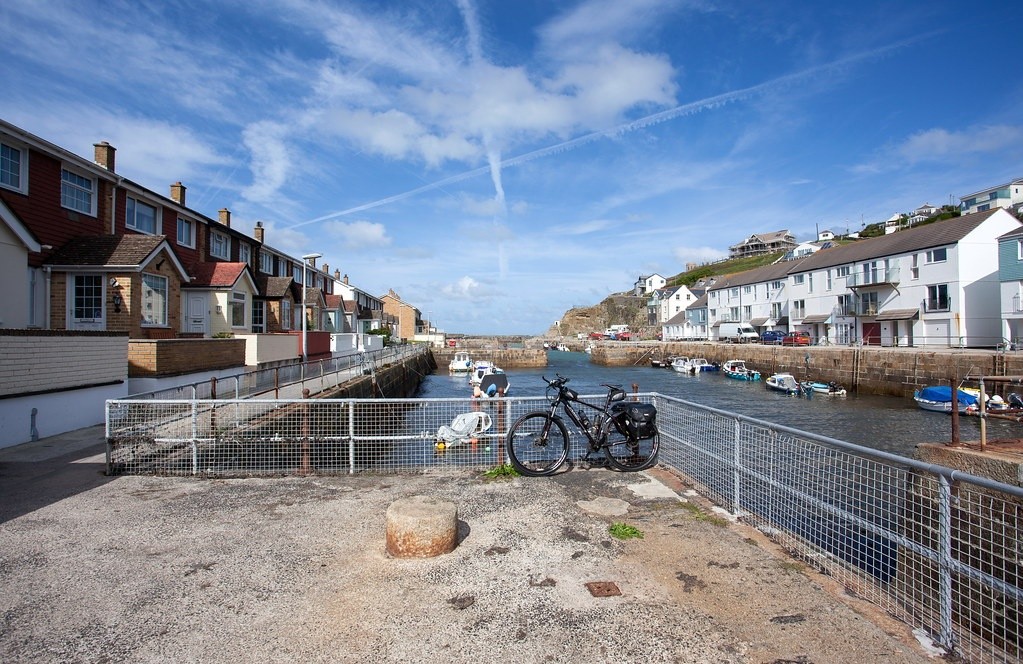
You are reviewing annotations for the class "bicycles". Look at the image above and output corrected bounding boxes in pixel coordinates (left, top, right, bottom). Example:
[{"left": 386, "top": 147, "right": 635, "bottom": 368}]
[{"left": 507, "top": 376, "right": 659, "bottom": 476}]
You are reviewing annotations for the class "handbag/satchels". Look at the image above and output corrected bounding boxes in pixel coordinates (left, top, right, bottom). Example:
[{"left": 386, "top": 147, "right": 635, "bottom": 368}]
[
  {"left": 626, "top": 403, "right": 658, "bottom": 442},
  {"left": 610, "top": 401, "right": 641, "bottom": 436}
]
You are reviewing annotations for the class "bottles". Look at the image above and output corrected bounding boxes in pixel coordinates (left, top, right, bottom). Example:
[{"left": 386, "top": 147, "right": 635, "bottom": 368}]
[
  {"left": 591, "top": 414, "right": 601, "bottom": 437},
  {"left": 577, "top": 409, "right": 591, "bottom": 430}
]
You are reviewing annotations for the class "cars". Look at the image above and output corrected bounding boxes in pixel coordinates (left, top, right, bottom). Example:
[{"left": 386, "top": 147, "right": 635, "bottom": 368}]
[
  {"left": 780, "top": 332, "right": 812, "bottom": 346},
  {"left": 760, "top": 331, "right": 787, "bottom": 345}
]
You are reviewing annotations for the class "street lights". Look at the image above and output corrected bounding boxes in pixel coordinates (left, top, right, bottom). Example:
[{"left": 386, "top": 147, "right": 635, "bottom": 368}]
[{"left": 303, "top": 253, "right": 322, "bottom": 360}]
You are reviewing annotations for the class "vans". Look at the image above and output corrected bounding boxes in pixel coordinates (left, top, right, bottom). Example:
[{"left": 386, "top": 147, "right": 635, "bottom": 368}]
[{"left": 718, "top": 323, "right": 759, "bottom": 343}]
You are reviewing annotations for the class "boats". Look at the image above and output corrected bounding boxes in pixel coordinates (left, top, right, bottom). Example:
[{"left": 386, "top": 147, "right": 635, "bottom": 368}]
[
  {"left": 475, "top": 368, "right": 509, "bottom": 407},
  {"left": 690, "top": 358, "right": 720, "bottom": 372},
  {"left": 766, "top": 372, "right": 801, "bottom": 396},
  {"left": 723, "top": 359, "right": 761, "bottom": 381},
  {"left": 648, "top": 357, "right": 671, "bottom": 369},
  {"left": 809, "top": 381, "right": 847, "bottom": 394},
  {"left": 469, "top": 360, "right": 503, "bottom": 387},
  {"left": 671, "top": 356, "right": 700, "bottom": 374},
  {"left": 448, "top": 351, "right": 473, "bottom": 371},
  {"left": 913, "top": 386, "right": 990, "bottom": 414}
]
